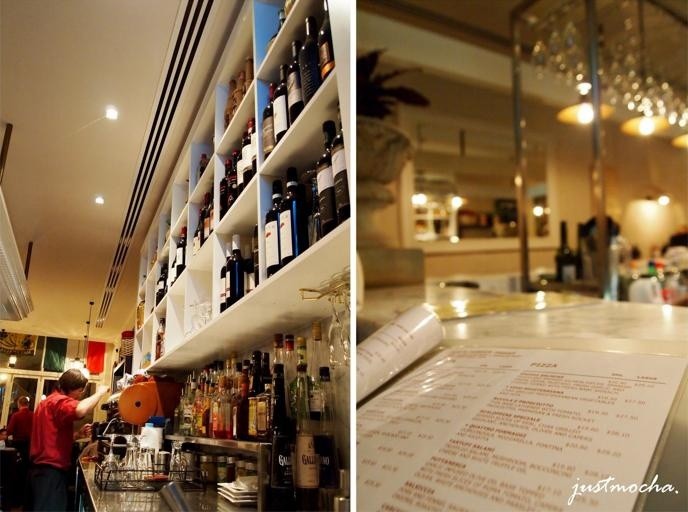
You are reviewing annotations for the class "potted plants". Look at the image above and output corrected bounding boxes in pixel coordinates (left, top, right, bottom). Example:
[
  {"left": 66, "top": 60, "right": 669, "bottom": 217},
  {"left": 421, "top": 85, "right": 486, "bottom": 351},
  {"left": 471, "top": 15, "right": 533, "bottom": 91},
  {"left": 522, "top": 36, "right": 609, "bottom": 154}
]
[{"left": 356, "top": 48, "right": 430, "bottom": 288}]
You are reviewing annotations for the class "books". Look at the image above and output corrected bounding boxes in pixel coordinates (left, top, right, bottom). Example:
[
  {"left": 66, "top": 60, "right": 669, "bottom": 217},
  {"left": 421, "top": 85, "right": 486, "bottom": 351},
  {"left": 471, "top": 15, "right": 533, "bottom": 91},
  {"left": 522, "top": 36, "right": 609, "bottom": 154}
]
[{"left": 357, "top": 301, "right": 688, "bottom": 511}]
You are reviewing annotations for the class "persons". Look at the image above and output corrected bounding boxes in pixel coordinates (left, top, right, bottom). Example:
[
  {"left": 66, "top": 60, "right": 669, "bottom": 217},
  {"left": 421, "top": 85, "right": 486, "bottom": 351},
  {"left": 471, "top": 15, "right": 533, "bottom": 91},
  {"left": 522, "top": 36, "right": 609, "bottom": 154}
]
[
  {"left": 29, "top": 367, "right": 111, "bottom": 512},
  {"left": 6, "top": 397, "right": 36, "bottom": 508},
  {"left": 0, "top": 428, "right": 13, "bottom": 448}
]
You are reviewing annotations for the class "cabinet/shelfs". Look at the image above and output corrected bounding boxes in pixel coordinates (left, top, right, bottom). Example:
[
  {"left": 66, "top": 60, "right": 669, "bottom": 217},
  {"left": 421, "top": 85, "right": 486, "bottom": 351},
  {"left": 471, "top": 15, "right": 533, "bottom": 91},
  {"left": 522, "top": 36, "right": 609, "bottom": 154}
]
[{"left": 111, "top": 0, "right": 351, "bottom": 403}]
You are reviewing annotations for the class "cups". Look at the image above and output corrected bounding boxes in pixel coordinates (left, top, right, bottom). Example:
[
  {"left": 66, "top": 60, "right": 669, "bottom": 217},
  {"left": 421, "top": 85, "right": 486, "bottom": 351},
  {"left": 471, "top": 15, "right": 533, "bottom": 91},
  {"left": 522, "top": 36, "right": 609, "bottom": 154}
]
[{"left": 123, "top": 445, "right": 153, "bottom": 470}]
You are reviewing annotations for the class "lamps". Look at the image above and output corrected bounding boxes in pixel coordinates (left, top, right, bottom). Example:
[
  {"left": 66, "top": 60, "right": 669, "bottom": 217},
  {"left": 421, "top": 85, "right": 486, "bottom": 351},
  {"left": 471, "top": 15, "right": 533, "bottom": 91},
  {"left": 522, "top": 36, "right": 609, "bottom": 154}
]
[
  {"left": 9, "top": 334, "right": 17, "bottom": 368},
  {"left": 557, "top": 0, "right": 688, "bottom": 149}
]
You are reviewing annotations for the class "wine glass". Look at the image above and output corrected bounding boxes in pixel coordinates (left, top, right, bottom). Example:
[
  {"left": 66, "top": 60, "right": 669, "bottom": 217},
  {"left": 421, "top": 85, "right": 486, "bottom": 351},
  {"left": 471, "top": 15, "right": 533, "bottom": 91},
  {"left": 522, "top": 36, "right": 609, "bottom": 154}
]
[
  {"left": 528, "top": 5, "right": 687, "bottom": 126},
  {"left": 328, "top": 282, "right": 351, "bottom": 369}
]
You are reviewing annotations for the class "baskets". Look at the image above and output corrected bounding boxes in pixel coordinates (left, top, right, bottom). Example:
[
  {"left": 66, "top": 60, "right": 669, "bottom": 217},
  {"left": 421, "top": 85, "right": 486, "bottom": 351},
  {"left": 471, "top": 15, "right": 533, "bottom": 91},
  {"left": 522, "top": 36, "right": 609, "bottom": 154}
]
[{"left": 118, "top": 337, "right": 135, "bottom": 357}]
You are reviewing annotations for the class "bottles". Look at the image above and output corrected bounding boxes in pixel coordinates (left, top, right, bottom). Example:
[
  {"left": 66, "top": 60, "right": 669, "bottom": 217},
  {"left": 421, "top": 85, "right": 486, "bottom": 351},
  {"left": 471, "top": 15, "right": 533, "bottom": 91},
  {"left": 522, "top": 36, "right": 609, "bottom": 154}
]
[
  {"left": 154, "top": 152, "right": 211, "bottom": 362},
  {"left": 556, "top": 221, "right": 578, "bottom": 281},
  {"left": 221, "top": 2, "right": 349, "bottom": 312},
  {"left": 174, "top": 321, "right": 336, "bottom": 508}
]
